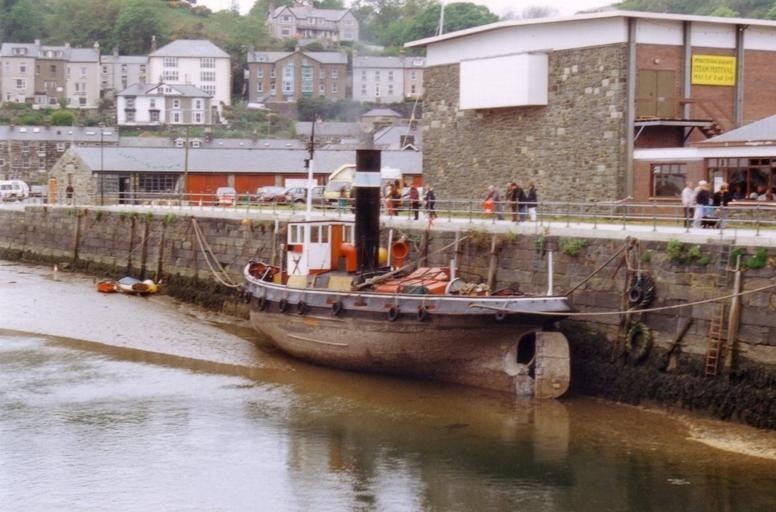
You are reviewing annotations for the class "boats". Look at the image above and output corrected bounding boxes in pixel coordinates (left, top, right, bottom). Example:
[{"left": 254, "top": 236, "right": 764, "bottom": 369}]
[
  {"left": 98, "top": 275, "right": 159, "bottom": 295},
  {"left": 241, "top": 149, "right": 574, "bottom": 397}
]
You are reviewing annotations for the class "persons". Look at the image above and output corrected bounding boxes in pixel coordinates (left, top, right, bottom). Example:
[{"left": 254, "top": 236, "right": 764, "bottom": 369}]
[
  {"left": 381, "top": 178, "right": 403, "bottom": 217},
  {"left": 680, "top": 179, "right": 734, "bottom": 235},
  {"left": 731, "top": 184, "right": 746, "bottom": 199},
  {"left": 407, "top": 182, "right": 421, "bottom": 221},
  {"left": 505, "top": 182, "right": 518, "bottom": 222},
  {"left": 421, "top": 184, "right": 438, "bottom": 220},
  {"left": 337, "top": 185, "right": 349, "bottom": 214},
  {"left": 348, "top": 186, "right": 358, "bottom": 214},
  {"left": 483, "top": 185, "right": 505, "bottom": 221},
  {"left": 506, "top": 183, "right": 528, "bottom": 223},
  {"left": 525, "top": 180, "right": 540, "bottom": 222},
  {"left": 755, "top": 183, "right": 768, "bottom": 196}
]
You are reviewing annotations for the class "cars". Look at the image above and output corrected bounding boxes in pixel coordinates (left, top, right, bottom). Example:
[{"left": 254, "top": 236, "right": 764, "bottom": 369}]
[
  {"left": 214, "top": 165, "right": 425, "bottom": 209},
  {"left": 1, "top": 179, "right": 47, "bottom": 202}
]
